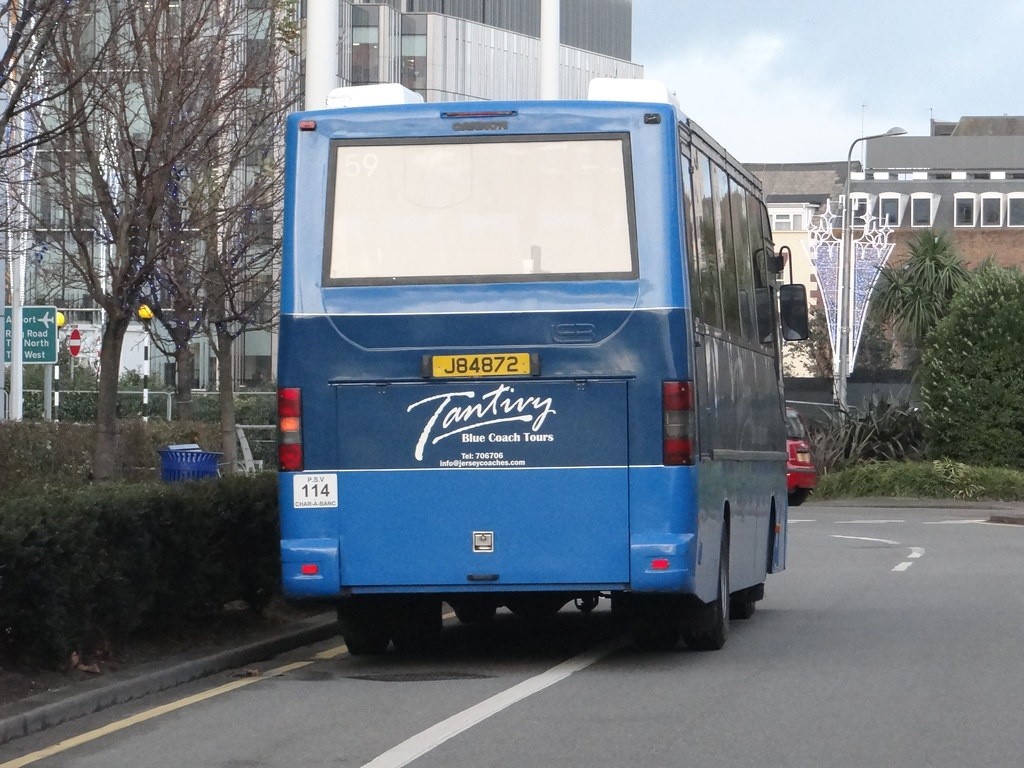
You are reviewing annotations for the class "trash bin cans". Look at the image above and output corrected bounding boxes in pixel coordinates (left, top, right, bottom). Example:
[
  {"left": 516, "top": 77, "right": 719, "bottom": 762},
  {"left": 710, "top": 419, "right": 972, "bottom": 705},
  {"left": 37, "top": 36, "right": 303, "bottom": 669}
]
[{"left": 154, "top": 448, "right": 224, "bottom": 484}]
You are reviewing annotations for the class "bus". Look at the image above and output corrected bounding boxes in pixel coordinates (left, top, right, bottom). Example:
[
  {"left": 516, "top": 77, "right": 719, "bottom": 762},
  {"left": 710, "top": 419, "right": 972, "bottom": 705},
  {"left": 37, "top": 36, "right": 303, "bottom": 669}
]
[{"left": 278, "top": 84, "right": 808, "bottom": 650}]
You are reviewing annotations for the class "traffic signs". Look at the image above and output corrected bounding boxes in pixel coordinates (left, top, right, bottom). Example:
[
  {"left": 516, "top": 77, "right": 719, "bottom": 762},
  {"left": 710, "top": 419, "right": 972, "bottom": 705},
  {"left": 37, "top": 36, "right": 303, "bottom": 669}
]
[{"left": 2, "top": 307, "right": 57, "bottom": 363}]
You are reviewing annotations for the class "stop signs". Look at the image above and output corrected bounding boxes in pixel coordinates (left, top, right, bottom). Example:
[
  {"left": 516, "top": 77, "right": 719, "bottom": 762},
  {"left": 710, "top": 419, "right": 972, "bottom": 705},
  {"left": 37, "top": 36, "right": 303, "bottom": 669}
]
[{"left": 69, "top": 329, "right": 81, "bottom": 356}]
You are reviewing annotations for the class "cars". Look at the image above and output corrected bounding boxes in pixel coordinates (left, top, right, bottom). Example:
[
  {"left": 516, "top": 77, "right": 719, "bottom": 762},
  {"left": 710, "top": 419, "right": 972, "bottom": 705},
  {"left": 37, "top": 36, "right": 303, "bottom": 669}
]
[{"left": 777, "top": 437, "right": 816, "bottom": 505}]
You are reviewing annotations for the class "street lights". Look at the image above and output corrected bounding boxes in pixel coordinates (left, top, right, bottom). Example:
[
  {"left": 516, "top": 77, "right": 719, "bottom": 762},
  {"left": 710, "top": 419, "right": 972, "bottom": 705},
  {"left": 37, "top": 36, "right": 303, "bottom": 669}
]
[
  {"left": 838, "top": 126, "right": 909, "bottom": 432},
  {"left": 54, "top": 312, "right": 65, "bottom": 425},
  {"left": 138, "top": 303, "right": 154, "bottom": 424}
]
